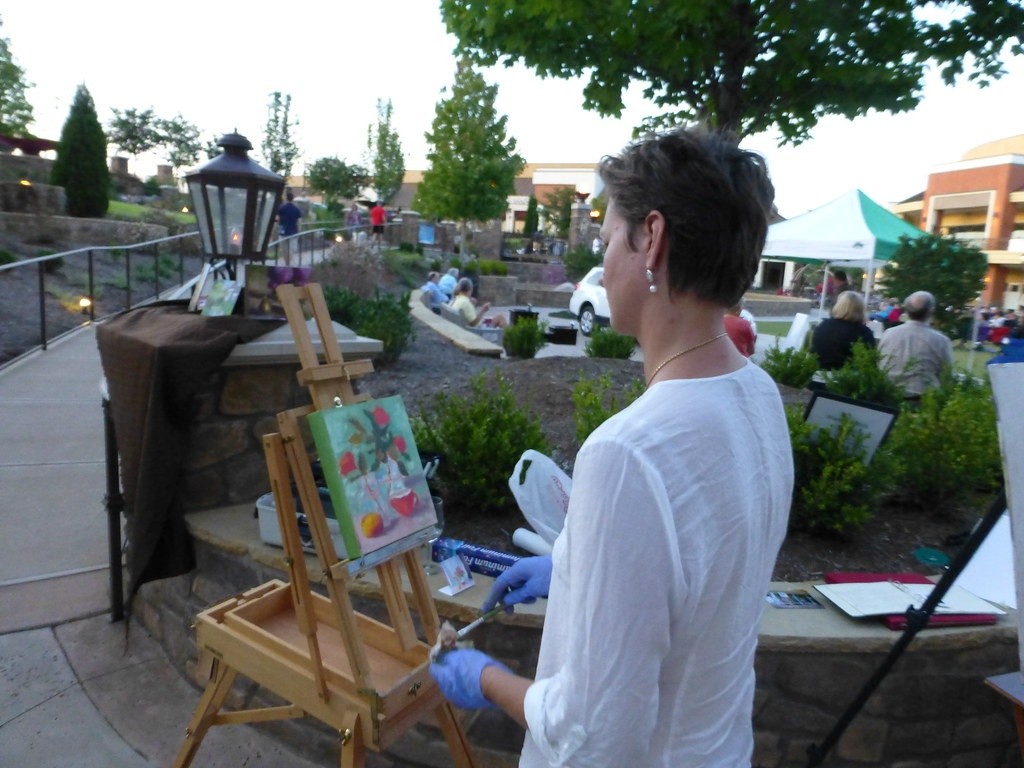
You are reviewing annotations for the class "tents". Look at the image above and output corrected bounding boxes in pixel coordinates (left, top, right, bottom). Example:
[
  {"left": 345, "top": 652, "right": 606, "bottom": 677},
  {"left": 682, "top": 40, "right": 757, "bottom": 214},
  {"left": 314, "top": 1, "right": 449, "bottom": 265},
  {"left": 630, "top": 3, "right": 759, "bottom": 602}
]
[{"left": 762, "top": 189, "right": 982, "bottom": 371}]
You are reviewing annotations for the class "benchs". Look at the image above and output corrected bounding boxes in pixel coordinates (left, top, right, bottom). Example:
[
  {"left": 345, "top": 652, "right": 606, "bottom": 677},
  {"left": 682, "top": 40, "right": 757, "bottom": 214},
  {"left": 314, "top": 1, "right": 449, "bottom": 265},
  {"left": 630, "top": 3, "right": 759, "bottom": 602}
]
[
  {"left": 421, "top": 288, "right": 444, "bottom": 315},
  {"left": 440, "top": 301, "right": 504, "bottom": 344}
]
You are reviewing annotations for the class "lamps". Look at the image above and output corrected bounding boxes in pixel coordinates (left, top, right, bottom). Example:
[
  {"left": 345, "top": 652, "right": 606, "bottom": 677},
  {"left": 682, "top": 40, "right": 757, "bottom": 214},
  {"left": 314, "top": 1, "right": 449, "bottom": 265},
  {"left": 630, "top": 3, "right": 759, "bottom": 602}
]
[
  {"left": 184, "top": 129, "right": 287, "bottom": 274},
  {"left": 80, "top": 298, "right": 91, "bottom": 313}
]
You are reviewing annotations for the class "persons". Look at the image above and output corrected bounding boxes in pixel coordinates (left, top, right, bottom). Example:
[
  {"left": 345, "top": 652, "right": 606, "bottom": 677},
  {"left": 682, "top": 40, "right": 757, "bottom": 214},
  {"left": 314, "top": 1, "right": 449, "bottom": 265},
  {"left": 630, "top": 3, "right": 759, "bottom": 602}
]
[
  {"left": 422, "top": 268, "right": 507, "bottom": 337},
  {"left": 349, "top": 204, "right": 361, "bottom": 240},
  {"left": 370, "top": 201, "right": 386, "bottom": 250},
  {"left": 980, "top": 305, "right": 1024, "bottom": 341},
  {"left": 275, "top": 193, "right": 302, "bottom": 266},
  {"left": 810, "top": 272, "right": 876, "bottom": 372},
  {"left": 725, "top": 300, "right": 755, "bottom": 358},
  {"left": 877, "top": 292, "right": 953, "bottom": 410},
  {"left": 868, "top": 298, "right": 902, "bottom": 323},
  {"left": 431, "top": 130, "right": 795, "bottom": 768}
]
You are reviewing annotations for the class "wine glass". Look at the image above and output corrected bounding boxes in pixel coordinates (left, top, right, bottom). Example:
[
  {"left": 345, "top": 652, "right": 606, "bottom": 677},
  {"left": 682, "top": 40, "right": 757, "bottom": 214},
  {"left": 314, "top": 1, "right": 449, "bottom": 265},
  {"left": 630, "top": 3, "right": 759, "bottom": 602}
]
[{"left": 419, "top": 496, "right": 444, "bottom": 576}]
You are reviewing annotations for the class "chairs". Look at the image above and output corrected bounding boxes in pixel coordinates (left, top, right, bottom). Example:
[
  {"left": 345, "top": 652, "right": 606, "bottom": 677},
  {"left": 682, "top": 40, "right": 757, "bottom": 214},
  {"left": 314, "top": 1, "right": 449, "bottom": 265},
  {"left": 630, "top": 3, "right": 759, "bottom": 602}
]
[
  {"left": 992, "top": 326, "right": 1010, "bottom": 343},
  {"left": 972, "top": 326, "right": 990, "bottom": 351}
]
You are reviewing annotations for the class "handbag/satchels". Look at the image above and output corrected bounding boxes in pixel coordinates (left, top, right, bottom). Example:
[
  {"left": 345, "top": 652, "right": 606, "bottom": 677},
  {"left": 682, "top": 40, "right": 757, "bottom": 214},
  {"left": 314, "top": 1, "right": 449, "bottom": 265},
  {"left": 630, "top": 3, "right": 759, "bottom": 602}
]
[{"left": 506, "top": 449, "right": 569, "bottom": 545}]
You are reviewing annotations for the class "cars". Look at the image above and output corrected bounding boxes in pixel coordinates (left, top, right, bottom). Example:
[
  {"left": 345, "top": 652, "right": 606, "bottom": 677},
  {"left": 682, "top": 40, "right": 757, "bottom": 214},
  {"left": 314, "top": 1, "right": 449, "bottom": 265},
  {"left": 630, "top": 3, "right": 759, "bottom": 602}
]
[{"left": 569, "top": 266, "right": 757, "bottom": 336}]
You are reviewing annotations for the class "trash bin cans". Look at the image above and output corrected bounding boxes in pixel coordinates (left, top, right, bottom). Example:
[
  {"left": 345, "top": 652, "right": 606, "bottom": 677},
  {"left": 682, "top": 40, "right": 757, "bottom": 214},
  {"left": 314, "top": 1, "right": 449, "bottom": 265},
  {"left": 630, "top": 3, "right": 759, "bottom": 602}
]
[{"left": 509, "top": 309, "right": 539, "bottom": 325}]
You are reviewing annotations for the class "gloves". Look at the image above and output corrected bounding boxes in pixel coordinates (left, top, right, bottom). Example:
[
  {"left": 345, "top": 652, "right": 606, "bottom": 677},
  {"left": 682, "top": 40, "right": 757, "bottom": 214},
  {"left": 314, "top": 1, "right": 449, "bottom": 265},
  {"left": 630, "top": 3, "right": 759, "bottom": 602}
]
[
  {"left": 429, "top": 649, "right": 514, "bottom": 710},
  {"left": 482, "top": 556, "right": 552, "bottom": 614}
]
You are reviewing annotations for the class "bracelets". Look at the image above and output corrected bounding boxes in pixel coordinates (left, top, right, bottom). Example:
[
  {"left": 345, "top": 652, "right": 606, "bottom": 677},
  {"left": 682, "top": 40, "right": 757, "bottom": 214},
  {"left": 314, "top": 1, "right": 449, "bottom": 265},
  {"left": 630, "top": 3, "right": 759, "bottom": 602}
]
[{"left": 646, "top": 332, "right": 728, "bottom": 387}]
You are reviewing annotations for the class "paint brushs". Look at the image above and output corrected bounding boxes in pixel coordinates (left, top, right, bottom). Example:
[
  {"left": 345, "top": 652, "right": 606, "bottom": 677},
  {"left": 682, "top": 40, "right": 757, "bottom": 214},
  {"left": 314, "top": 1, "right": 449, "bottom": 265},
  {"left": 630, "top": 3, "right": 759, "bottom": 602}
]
[{"left": 456, "top": 605, "right": 506, "bottom": 638}]
